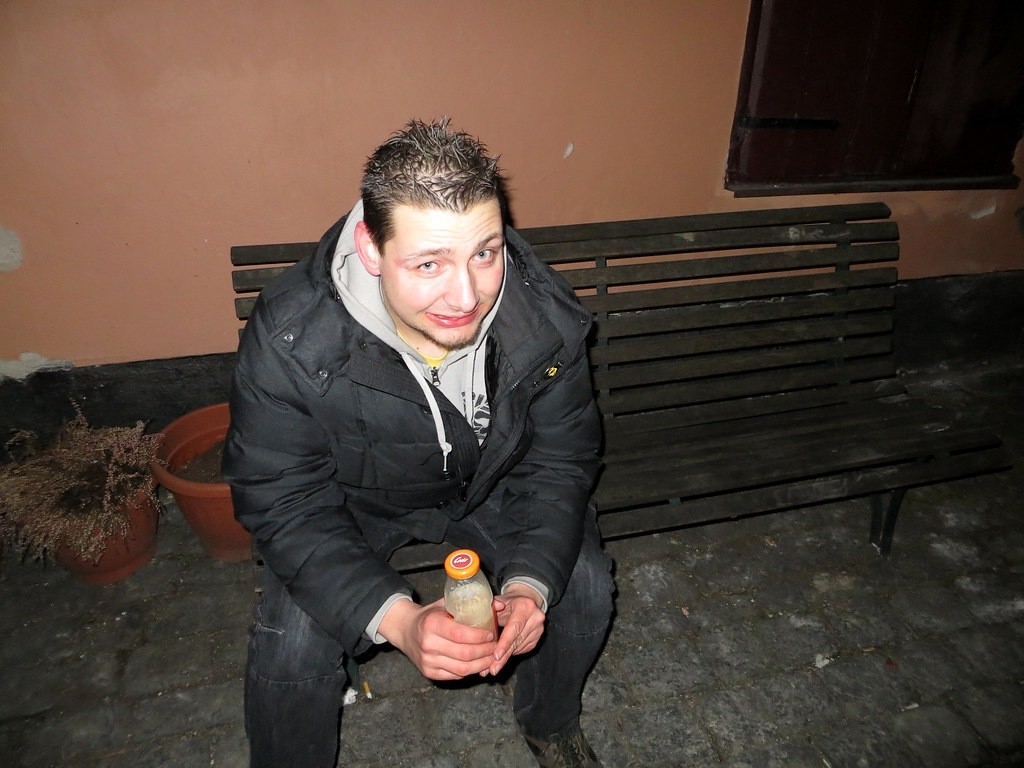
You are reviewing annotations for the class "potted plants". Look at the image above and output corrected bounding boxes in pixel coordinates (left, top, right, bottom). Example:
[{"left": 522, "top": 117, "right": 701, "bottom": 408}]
[{"left": 0, "top": 395, "right": 165, "bottom": 585}]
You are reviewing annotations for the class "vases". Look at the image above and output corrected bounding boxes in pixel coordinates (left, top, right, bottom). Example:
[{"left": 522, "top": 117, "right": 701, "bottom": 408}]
[{"left": 149, "top": 403, "right": 253, "bottom": 563}]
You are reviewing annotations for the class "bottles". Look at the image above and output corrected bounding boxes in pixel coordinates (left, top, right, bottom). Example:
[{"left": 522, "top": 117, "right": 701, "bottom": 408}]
[{"left": 443, "top": 549, "right": 500, "bottom": 643}]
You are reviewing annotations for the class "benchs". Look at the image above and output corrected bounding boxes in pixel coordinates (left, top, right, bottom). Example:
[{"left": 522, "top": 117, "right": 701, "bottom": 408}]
[{"left": 229, "top": 203, "right": 1015, "bottom": 717}]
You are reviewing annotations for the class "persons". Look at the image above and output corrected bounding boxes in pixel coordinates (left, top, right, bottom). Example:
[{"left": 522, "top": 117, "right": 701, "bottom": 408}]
[{"left": 221, "top": 113, "right": 614, "bottom": 768}]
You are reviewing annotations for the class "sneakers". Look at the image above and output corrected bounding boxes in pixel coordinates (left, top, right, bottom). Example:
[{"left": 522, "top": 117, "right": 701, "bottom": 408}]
[{"left": 520, "top": 723, "right": 604, "bottom": 768}]
